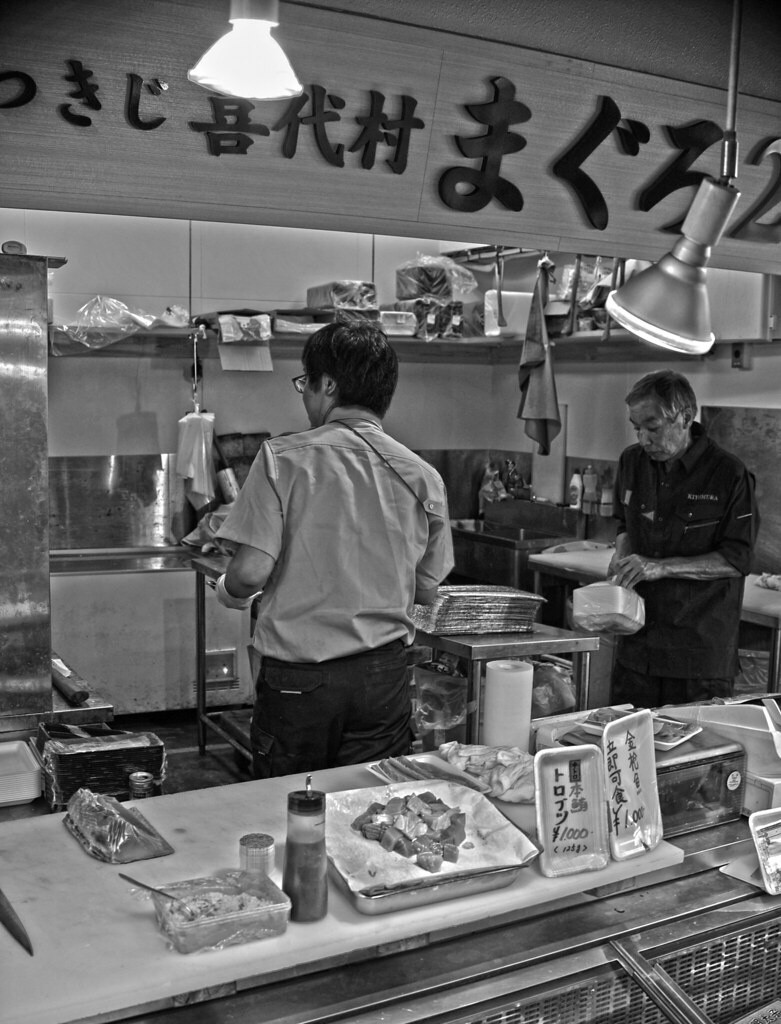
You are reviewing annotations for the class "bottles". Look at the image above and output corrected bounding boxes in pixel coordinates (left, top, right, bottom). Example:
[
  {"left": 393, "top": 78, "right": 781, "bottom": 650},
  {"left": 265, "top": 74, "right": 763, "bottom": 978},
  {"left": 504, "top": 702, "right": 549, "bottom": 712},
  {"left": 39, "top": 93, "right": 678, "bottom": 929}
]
[
  {"left": 568, "top": 468, "right": 582, "bottom": 510},
  {"left": 582, "top": 465, "right": 598, "bottom": 514},
  {"left": 600, "top": 465, "right": 614, "bottom": 517},
  {"left": 239, "top": 833, "right": 274, "bottom": 875},
  {"left": 281, "top": 773, "right": 328, "bottom": 923}
]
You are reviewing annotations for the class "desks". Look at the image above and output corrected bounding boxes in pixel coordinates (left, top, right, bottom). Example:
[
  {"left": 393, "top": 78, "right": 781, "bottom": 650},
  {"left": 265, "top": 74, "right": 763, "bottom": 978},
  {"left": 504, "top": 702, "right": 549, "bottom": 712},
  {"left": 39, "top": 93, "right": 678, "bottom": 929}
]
[
  {"left": 530, "top": 550, "right": 781, "bottom": 694},
  {"left": 192, "top": 555, "right": 601, "bottom": 760}
]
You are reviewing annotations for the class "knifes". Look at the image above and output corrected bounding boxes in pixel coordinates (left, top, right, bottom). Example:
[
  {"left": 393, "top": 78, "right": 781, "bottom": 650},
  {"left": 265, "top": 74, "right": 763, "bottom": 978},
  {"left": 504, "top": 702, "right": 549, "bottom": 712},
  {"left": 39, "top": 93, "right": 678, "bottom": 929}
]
[{"left": 0, "top": 888, "right": 36, "bottom": 958}]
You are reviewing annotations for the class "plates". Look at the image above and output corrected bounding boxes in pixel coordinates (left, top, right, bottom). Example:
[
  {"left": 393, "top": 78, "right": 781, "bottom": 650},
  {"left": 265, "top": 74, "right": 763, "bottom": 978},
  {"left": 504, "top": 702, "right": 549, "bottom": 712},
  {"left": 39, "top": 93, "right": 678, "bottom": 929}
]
[
  {"left": 1, "top": 722, "right": 170, "bottom": 807},
  {"left": 367, "top": 753, "right": 491, "bottom": 796}
]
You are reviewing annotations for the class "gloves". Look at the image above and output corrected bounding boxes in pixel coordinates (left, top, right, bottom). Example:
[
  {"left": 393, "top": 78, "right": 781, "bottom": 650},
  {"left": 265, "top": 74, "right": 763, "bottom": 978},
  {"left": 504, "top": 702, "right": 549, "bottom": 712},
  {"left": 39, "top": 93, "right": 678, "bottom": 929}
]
[{"left": 214, "top": 573, "right": 262, "bottom": 609}]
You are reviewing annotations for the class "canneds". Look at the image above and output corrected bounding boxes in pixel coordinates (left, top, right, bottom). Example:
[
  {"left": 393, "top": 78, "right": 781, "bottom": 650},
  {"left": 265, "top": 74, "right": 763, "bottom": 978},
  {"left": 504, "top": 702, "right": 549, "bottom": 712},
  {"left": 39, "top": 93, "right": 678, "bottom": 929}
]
[{"left": 129, "top": 771, "right": 154, "bottom": 800}]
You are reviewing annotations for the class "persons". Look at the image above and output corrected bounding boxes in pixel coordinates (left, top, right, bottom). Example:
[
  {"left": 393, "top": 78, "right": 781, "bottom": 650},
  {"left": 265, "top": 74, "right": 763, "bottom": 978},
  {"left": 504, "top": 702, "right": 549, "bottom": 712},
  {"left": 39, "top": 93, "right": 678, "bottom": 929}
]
[
  {"left": 214, "top": 321, "right": 453, "bottom": 777},
  {"left": 607, "top": 371, "right": 762, "bottom": 706}
]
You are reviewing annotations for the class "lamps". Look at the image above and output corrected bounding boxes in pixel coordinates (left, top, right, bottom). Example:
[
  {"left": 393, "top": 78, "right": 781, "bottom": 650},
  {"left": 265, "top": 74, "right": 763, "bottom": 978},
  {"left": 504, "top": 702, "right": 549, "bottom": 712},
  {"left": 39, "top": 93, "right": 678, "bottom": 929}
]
[
  {"left": 189, "top": 0, "right": 303, "bottom": 100},
  {"left": 606, "top": 0, "right": 743, "bottom": 354}
]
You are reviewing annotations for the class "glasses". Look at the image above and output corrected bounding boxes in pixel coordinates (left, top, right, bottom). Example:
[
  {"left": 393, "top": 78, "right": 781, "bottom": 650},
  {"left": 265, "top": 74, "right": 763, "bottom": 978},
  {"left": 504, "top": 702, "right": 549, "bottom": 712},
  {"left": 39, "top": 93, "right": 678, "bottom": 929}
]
[{"left": 292, "top": 372, "right": 314, "bottom": 392}]
